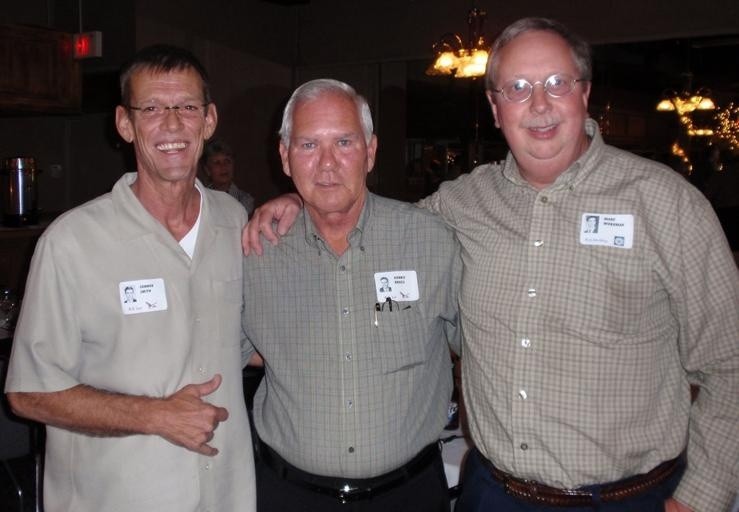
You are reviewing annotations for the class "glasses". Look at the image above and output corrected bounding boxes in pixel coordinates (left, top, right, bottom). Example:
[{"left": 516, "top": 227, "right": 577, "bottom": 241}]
[
  {"left": 128, "top": 102, "right": 209, "bottom": 123},
  {"left": 488, "top": 74, "right": 585, "bottom": 106}
]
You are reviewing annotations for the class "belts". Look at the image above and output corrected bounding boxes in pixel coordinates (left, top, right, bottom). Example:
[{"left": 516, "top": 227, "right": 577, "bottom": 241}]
[
  {"left": 481, "top": 456, "right": 688, "bottom": 509},
  {"left": 258, "top": 441, "right": 442, "bottom": 502}
]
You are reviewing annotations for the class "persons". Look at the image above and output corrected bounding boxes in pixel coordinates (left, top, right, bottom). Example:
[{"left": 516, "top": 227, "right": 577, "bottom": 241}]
[
  {"left": 3, "top": 44, "right": 263, "bottom": 512},
  {"left": 585, "top": 217, "right": 598, "bottom": 233},
  {"left": 240, "top": 16, "right": 739, "bottom": 512},
  {"left": 380, "top": 277, "right": 392, "bottom": 292},
  {"left": 200, "top": 138, "right": 256, "bottom": 218},
  {"left": 124, "top": 287, "right": 137, "bottom": 303},
  {"left": 241, "top": 79, "right": 462, "bottom": 512}
]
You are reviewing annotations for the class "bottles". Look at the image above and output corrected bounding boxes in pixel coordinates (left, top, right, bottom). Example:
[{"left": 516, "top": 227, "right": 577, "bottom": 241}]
[{"left": 4, "top": 157, "right": 35, "bottom": 227}]
